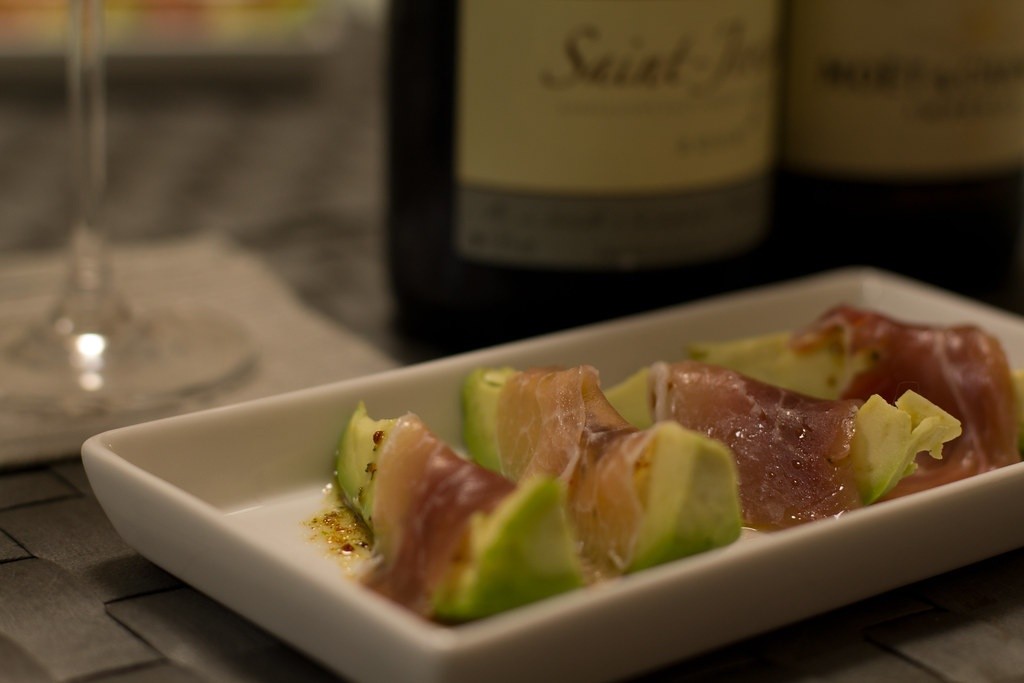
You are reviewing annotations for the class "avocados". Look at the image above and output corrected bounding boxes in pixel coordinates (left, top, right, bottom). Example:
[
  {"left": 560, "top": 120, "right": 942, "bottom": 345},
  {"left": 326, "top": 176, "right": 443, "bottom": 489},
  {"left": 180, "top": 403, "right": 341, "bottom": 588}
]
[{"left": 333, "top": 328, "right": 966, "bottom": 627}]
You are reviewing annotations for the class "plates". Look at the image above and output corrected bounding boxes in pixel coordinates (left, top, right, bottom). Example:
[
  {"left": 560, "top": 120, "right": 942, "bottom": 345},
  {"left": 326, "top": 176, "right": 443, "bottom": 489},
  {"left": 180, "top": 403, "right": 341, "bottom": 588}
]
[
  {"left": 2, "top": 233, "right": 402, "bottom": 470},
  {"left": 83, "top": 264, "right": 1024, "bottom": 682},
  {"left": 0, "top": 0, "right": 349, "bottom": 85}
]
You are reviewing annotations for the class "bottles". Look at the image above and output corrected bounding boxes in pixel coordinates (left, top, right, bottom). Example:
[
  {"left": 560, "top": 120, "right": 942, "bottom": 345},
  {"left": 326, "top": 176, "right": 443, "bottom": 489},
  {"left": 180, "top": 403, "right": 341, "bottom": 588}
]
[{"left": 380, "top": 0, "right": 1024, "bottom": 365}]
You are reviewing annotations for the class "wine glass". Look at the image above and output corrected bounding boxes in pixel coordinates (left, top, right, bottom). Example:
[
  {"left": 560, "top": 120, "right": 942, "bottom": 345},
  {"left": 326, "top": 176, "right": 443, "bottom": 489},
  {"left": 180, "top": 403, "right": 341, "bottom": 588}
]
[{"left": 1, "top": 1, "right": 263, "bottom": 413}]
